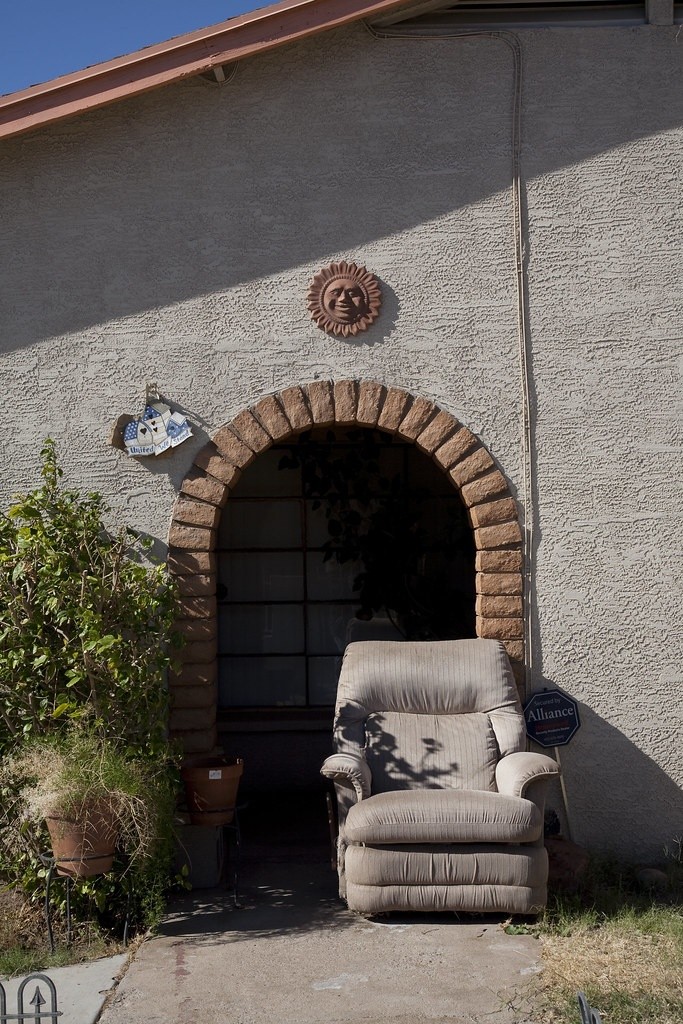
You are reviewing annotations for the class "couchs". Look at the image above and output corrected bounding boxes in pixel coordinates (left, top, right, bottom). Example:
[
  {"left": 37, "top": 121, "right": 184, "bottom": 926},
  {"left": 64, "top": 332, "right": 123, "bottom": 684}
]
[{"left": 326, "top": 639, "right": 559, "bottom": 923}]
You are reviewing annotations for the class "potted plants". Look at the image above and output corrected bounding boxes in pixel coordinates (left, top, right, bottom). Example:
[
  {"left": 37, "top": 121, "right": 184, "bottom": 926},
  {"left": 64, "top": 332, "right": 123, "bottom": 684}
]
[
  {"left": 178, "top": 752, "right": 245, "bottom": 825},
  {"left": 0, "top": 710, "right": 182, "bottom": 889}
]
